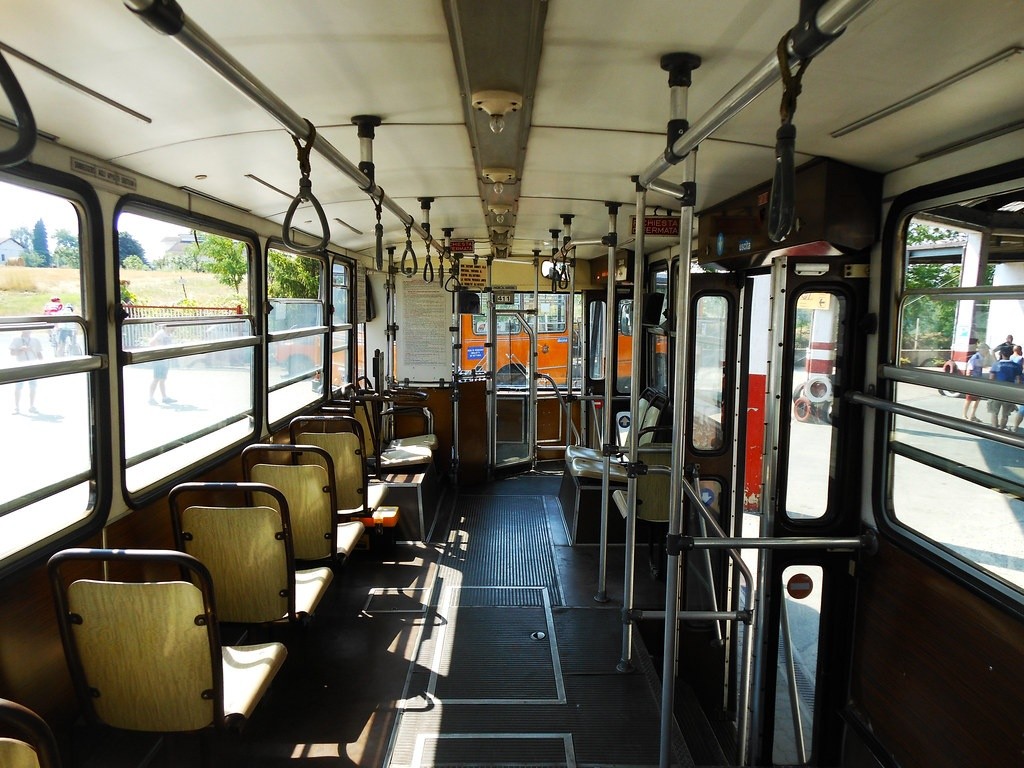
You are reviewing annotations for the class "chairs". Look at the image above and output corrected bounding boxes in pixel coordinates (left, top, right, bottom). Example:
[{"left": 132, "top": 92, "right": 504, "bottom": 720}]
[
  {"left": 565, "top": 387, "right": 674, "bottom": 584},
  {"left": 0, "top": 377, "right": 438, "bottom": 768}
]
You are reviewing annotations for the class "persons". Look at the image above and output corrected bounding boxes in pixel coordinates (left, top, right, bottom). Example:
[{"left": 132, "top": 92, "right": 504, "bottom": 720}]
[
  {"left": 9, "top": 329, "right": 43, "bottom": 415},
  {"left": 147, "top": 325, "right": 177, "bottom": 406},
  {"left": 962, "top": 342, "right": 990, "bottom": 424},
  {"left": 44, "top": 295, "right": 78, "bottom": 356},
  {"left": 986, "top": 334, "right": 1024, "bottom": 433}
]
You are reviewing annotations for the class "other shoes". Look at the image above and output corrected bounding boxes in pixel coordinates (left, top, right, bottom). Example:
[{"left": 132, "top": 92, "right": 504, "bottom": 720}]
[
  {"left": 970, "top": 417, "right": 982, "bottom": 422},
  {"left": 29, "top": 407, "right": 39, "bottom": 413},
  {"left": 11, "top": 410, "right": 20, "bottom": 415},
  {"left": 162, "top": 397, "right": 177, "bottom": 403},
  {"left": 149, "top": 399, "right": 159, "bottom": 406}
]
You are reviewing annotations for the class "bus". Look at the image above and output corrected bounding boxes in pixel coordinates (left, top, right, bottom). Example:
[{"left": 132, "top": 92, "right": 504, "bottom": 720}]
[{"left": 310, "top": 263, "right": 669, "bottom": 393}]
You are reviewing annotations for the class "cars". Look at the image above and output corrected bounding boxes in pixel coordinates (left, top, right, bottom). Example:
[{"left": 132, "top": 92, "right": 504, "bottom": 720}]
[{"left": 275, "top": 334, "right": 322, "bottom": 376}]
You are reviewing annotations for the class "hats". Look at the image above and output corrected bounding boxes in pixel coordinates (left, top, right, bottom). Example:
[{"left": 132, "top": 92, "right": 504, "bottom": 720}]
[{"left": 978, "top": 343, "right": 990, "bottom": 349}]
